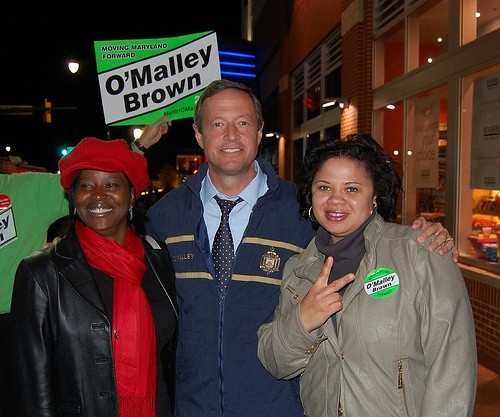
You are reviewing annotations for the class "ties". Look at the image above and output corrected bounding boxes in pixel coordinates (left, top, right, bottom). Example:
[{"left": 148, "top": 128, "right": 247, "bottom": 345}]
[{"left": 211, "top": 195, "right": 244, "bottom": 298}]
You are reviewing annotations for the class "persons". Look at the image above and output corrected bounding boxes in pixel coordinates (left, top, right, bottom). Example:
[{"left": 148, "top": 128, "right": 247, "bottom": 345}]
[
  {"left": 44, "top": 78, "right": 460, "bottom": 417},
  {"left": 254, "top": 134, "right": 477, "bottom": 417},
  {"left": 9, "top": 137, "right": 180, "bottom": 417},
  {"left": 0, "top": 115, "right": 173, "bottom": 417}
]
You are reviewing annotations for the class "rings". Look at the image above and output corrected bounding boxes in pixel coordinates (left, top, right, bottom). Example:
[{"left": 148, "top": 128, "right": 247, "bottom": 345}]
[{"left": 446, "top": 237, "right": 454, "bottom": 241}]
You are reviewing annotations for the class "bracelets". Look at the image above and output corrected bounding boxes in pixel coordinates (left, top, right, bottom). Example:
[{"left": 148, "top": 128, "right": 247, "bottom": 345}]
[{"left": 134, "top": 139, "right": 148, "bottom": 152}]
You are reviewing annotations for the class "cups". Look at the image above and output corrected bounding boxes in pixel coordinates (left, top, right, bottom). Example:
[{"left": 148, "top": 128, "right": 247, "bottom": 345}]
[{"left": 482, "top": 227, "right": 492, "bottom": 238}]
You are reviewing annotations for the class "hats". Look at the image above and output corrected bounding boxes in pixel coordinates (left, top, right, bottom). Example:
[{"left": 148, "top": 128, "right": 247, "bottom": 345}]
[{"left": 59, "top": 138, "right": 150, "bottom": 200}]
